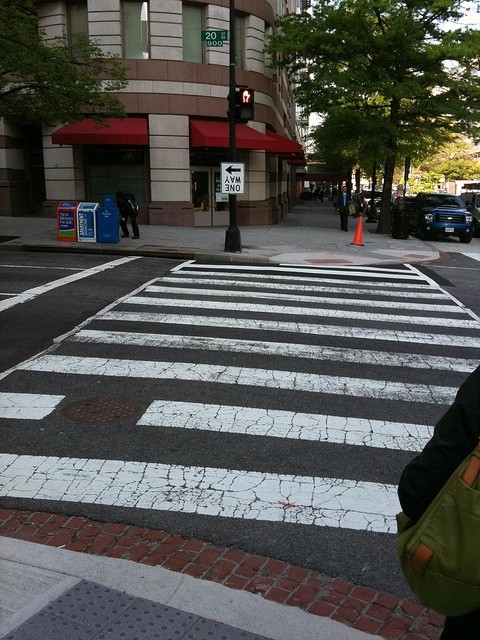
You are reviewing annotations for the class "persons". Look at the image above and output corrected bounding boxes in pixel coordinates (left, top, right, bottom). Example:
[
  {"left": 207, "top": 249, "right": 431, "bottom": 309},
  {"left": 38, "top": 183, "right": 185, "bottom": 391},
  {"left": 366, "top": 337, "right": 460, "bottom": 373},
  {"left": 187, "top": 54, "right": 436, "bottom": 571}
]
[
  {"left": 352, "top": 190, "right": 363, "bottom": 218},
  {"left": 398, "top": 364, "right": 479, "bottom": 640},
  {"left": 116, "top": 191, "right": 141, "bottom": 239},
  {"left": 311, "top": 184, "right": 328, "bottom": 203},
  {"left": 336, "top": 186, "right": 349, "bottom": 232}
]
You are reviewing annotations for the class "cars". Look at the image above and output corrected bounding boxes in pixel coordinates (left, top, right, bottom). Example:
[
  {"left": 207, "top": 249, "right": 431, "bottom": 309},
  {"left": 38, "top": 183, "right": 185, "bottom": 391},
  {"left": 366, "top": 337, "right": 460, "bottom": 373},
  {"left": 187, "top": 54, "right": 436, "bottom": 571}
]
[
  {"left": 392, "top": 198, "right": 417, "bottom": 225},
  {"left": 367, "top": 198, "right": 394, "bottom": 220}
]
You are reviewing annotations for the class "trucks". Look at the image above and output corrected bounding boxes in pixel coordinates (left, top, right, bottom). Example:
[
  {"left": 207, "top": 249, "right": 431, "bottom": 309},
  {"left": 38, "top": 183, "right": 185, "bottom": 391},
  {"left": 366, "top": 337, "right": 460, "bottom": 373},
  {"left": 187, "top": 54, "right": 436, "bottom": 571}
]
[{"left": 461, "top": 192, "right": 480, "bottom": 238}]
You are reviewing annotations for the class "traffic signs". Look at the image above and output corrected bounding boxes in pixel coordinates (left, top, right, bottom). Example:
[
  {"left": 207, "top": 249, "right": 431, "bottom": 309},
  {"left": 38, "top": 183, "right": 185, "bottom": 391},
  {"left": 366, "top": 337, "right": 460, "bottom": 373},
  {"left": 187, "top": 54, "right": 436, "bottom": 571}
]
[{"left": 198, "top": 28, "right": 229, "bottom": 50}]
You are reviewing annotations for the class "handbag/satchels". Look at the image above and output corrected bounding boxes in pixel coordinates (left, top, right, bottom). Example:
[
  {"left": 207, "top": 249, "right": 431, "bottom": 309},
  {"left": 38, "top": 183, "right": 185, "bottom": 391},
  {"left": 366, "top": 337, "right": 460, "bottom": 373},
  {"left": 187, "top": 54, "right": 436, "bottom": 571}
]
[
  {"left": 395, "top": 444, "right": 480, "bottom": 616},
  {"left": 348, "top": 200, "right": 356, "bottom": 215}
]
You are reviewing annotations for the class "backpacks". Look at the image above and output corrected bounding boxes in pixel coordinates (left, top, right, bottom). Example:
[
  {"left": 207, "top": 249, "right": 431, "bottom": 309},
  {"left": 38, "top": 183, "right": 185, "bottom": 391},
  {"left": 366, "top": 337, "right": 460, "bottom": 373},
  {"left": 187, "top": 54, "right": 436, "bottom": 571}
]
[{"left": 124, "top": 195, "right": 140, "bottom": 217}]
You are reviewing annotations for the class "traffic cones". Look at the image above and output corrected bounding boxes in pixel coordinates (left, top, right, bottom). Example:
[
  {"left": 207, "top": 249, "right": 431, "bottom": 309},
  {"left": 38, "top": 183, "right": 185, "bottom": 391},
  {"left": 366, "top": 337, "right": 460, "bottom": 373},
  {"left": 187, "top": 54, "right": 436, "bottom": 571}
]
[{"left": 350, "top": 215, "right": 365, "bottom": 246}]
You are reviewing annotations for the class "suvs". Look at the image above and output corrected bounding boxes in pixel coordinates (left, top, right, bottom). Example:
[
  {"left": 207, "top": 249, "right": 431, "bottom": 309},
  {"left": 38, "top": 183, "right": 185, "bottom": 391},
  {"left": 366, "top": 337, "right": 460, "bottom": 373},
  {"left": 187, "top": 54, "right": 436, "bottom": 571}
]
[
  {"left": 360, "top": 190, "right": 384, "bottom": 214},
  {"left": 408, "top": 191, "right": 475, "bottom": 244}
]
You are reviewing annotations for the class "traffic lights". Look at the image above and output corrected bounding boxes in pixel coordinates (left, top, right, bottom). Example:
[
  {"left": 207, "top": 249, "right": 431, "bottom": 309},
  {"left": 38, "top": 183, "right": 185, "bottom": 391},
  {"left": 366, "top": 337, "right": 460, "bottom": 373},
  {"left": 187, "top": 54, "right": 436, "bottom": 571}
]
[{"left": 226, "top": 85, "right": 255, "bottom": 124}]
